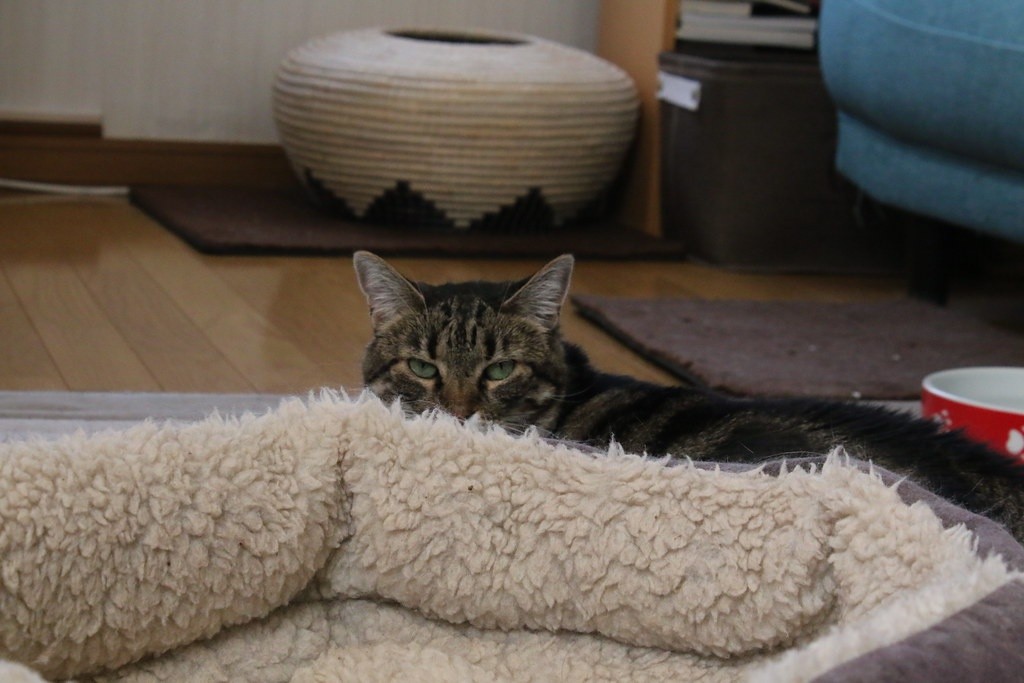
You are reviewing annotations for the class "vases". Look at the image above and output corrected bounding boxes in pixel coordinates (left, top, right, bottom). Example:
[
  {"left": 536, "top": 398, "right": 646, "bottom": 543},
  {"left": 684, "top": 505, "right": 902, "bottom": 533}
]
[{"left": 275, "top": 27, "right": 642, "bottom": 233}]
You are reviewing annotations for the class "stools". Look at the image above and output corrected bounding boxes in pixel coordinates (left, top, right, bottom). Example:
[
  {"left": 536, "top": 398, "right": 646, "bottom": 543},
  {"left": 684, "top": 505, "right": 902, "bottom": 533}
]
[{"left": 818, "top": 0, "right": 1024, "bottom": 306}]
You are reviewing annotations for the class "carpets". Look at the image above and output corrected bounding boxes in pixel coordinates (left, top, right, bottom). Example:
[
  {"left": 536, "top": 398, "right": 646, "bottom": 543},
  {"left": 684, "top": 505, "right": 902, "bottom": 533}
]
[
  {"left": 570, "top": 291, "right": 1024, "bottom": 401},
  {"left": 132, "top": 186, "right": 691, "bottom": 262}
]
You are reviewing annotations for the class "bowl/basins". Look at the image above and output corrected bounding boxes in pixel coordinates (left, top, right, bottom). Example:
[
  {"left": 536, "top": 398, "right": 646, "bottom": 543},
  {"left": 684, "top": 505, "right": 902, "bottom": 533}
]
[{"left": 919, "top": 364, "right": 1024, "bottom": 467}]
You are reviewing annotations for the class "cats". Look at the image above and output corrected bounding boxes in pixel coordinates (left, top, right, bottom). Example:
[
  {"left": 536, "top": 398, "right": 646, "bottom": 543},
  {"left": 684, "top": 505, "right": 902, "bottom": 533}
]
[{"left": 351, "top": 249, "right": 1024, "bottom": 550}]
